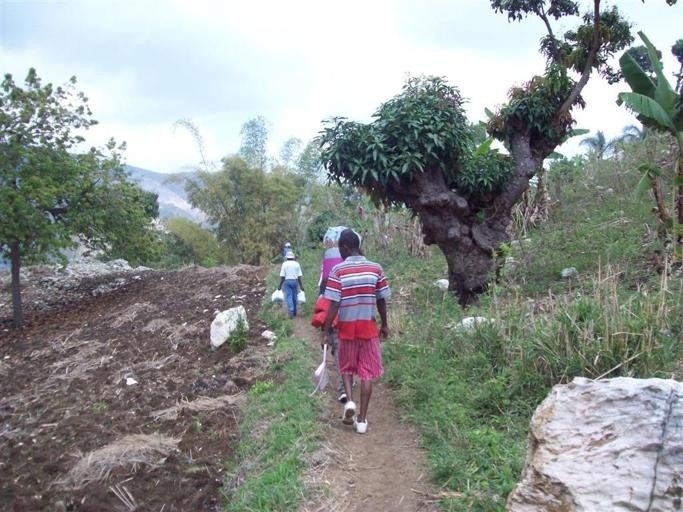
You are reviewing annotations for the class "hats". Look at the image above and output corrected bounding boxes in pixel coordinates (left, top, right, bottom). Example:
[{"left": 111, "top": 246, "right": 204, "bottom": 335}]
[
  {"left": 285, "top": 242, "right": 291, "bottom": 246},
  {"left": 285, "top": 252, "right": 295, "bottom": 258}
]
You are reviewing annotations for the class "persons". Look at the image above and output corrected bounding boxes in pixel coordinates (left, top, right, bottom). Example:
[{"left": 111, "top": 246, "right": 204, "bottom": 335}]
[
  {"left": 278, "top": 242, "right": 305, "bottom": 319},
  {"left": 311, "top": 226, "right": 391, "bottom": 434}
]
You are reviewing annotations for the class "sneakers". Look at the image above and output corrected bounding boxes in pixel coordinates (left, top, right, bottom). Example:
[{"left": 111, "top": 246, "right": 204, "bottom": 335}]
[
  {"left": 353, "top": 418, "right": 368, "bottom": 433},
  {"left": 338, "top": 382, "right": 355, "bottom": 401},
  {"left": 342, "top": 401, "right": 356, "bottom": 426}
]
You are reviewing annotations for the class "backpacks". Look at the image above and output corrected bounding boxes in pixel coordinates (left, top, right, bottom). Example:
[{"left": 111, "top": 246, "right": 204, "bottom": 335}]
[{"left": 323, "top": 247, "right": 343, "bottom": 286}]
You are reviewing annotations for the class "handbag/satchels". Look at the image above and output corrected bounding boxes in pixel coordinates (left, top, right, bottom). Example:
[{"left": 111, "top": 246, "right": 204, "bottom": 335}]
[{"left": 311, "top": 294, "right": 339, "bottom": 328}]
